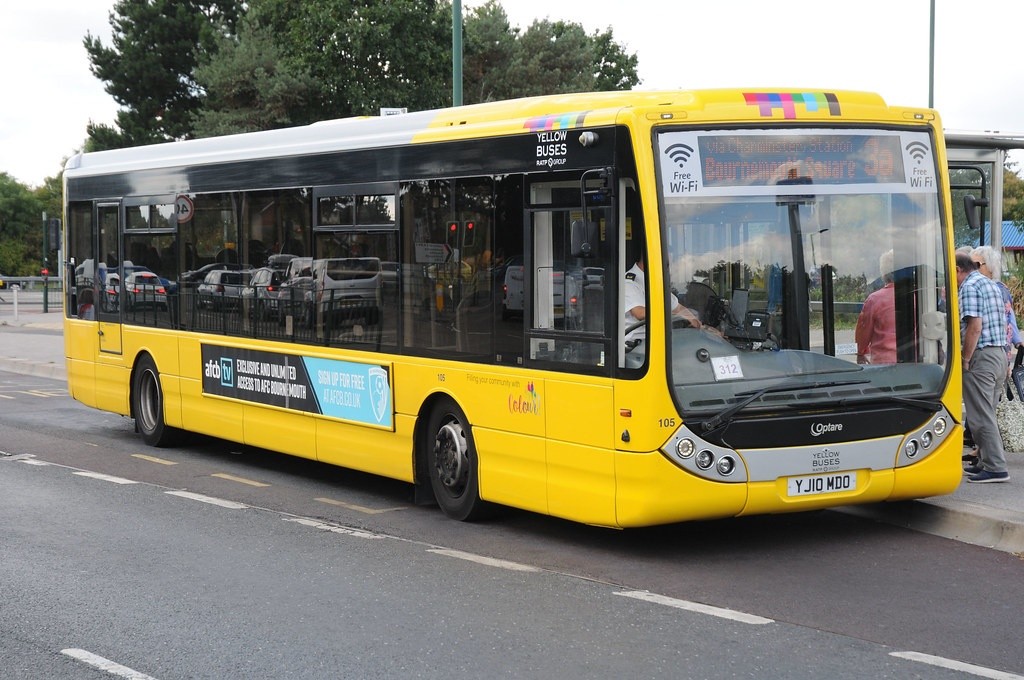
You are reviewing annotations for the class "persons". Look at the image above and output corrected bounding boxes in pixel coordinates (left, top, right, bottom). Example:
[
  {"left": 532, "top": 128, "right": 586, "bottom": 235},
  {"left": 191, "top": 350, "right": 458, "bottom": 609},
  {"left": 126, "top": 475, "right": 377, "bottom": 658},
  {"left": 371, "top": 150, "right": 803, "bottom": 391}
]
[
  {"left": 939, "top": 245, "right": 1024, "bottom": 483},
  {"left": 853, "top": 249, "right": 917, "bottom": 366},
  {"left": 624, "top": 249, "right": 702, "bottom": 345}
]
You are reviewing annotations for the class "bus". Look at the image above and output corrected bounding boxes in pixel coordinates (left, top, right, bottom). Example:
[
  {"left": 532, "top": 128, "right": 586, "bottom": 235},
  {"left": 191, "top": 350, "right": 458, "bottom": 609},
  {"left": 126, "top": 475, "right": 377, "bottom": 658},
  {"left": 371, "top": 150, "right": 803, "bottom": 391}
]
[{"left": 55, "top": 85, "right": 969, "bottom": 536}]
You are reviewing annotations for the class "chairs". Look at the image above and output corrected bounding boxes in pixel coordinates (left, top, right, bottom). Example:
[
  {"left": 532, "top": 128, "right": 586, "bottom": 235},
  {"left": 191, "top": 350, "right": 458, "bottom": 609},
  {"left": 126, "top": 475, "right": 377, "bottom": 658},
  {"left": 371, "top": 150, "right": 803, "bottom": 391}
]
[
  {"left": 567, "top": 288, "right": 604, "bottom": 332},
  {"left": 680, "top": 283, "right": 719, "bottom": 330}
]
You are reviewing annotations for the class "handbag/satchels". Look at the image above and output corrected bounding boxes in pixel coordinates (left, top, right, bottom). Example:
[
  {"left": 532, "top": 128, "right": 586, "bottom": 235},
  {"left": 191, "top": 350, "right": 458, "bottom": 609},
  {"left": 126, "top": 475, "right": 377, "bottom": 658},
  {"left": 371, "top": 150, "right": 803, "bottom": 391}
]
[
  {"left": 1006, "top": 346, "right": 1024, "bottom": 403},
  {"left": 996, "top": 378, "right": 1024, "bottom": 452}
]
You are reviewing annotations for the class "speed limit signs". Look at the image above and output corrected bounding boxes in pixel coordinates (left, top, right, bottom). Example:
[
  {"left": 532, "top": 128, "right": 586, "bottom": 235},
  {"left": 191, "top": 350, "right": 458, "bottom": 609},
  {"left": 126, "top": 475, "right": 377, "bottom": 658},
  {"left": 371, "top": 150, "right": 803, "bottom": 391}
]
[{"left": 174, "top": 195, "right": 195, "bottom": 224}]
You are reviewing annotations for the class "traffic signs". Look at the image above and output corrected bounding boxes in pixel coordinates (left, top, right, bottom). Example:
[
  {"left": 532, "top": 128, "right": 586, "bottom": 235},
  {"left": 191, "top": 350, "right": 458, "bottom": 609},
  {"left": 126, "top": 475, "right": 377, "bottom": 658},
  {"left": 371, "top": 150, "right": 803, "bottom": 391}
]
[{"left": 415, "top": 242, "right": 454, "bottom": 265}]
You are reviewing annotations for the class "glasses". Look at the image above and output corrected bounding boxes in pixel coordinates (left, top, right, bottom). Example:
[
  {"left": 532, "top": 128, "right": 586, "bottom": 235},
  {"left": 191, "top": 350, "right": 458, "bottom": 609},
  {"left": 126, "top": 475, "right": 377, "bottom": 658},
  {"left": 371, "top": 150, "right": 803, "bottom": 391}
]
[{"left": 974, "top": 262, "right": 986, "bottom": 269}]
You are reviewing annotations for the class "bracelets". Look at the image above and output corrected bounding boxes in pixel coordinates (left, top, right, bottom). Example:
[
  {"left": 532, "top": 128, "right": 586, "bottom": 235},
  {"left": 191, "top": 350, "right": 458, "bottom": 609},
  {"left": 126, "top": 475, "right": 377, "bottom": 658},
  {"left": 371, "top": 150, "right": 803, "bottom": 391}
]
[{"left": 961, "top": 355, "right": 970, "bottom": 362}]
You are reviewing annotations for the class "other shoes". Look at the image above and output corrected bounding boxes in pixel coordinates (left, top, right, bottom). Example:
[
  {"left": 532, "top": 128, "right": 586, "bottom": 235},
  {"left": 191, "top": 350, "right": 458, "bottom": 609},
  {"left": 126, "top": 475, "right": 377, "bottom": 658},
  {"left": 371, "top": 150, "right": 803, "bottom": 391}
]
[
  {"left": 961, "top": 454, "right": 979, "bottom": 465},
  {"left": 963, "top": 465, "right": 982, "bottom": 475},
  {"left": 967, "top": 470, "right": 1010, "bottom": 482}
]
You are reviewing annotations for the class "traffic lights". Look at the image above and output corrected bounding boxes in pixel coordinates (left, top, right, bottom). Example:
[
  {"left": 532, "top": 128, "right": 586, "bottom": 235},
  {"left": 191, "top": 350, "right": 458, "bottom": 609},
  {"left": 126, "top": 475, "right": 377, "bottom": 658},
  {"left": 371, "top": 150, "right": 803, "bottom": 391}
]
[
  {"left": 445, "top": 221, "right": 460, "bottom": 249},
  {"left": 462, "top": 221, "right": 475, "bottom": 248}
]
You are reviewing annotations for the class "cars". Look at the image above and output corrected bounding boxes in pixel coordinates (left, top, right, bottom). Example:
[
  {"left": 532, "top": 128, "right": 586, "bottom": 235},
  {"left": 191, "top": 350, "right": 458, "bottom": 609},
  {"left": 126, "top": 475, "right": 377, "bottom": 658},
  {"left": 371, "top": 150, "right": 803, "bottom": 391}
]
[
  {"left": 420, "top": 258, "right": 605, "bottom": 328},
  {"left": 0, "top": 273, "right": 26, "bottom": 291},
  {"left": 74, "top": 254, "right": 401, "bottom": 329}
]
